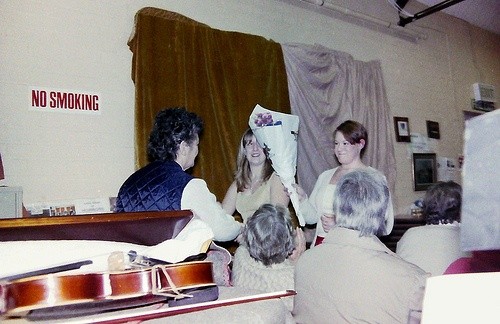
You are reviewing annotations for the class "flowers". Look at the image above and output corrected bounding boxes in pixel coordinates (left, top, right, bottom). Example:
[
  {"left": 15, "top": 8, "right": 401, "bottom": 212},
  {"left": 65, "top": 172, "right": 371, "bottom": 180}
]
[{"left": 250, "top": 111, "right": 282, "bottom": 127}]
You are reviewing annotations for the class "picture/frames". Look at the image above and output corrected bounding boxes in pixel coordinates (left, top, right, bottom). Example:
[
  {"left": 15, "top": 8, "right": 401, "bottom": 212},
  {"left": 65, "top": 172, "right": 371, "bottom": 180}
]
[
  {"left": 412, "top": 152, "right": 438, "bottom": 192},
  {"left": 425, "top": 120, "right": 441, "bottom": 140},
  {"left": 393, "top": 116, "right": 411, "bottom": 143}
]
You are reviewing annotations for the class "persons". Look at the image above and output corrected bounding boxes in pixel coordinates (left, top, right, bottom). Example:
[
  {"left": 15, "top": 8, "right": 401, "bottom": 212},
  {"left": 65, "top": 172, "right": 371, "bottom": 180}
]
[
  {"left": 115, "top": 107, "right": 243, "bottom": 284},
  {"left": 216, "top": 126, "right": 290, "bottom": 223},
  {"left": 295, "top": 168, "right": 429, "bottom": 324},
  {"left": 232, "top": 204, "right": 306, "bottom": 313},
  {"left": 396, "top": 180, "right": 471, "bottom": 274},
  {"left": 283, "top": 120, "right": 394, "bottom": 247}
]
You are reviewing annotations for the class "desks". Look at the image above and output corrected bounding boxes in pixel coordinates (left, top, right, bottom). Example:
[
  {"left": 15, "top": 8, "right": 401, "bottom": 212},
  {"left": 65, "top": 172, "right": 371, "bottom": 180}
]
[{"left": 377, "top": 213, "right": 427, "bottom": 255}]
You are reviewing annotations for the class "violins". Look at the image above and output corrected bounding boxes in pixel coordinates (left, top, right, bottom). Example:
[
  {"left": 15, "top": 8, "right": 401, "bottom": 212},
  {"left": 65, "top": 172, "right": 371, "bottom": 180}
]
[{"left": 0, "top": 260, "right": 218, "bottom": 317}]
